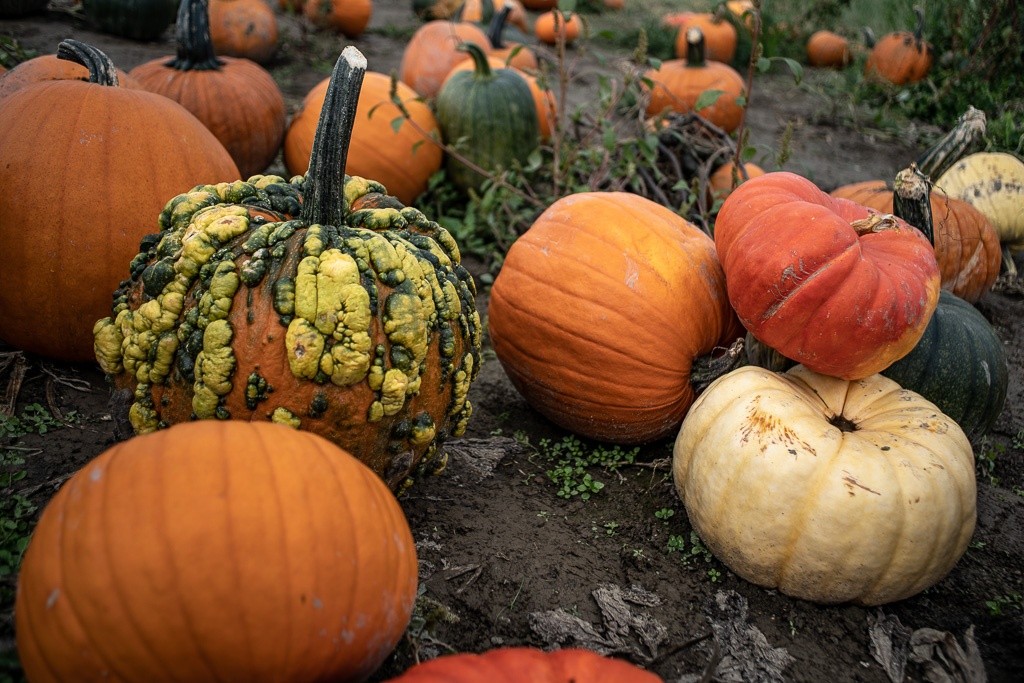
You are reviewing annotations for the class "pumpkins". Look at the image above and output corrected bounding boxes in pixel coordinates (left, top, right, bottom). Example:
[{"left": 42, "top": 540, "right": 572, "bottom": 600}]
[{"left": 0, "top": 0, "right": 1024, "bottom": 683}]
